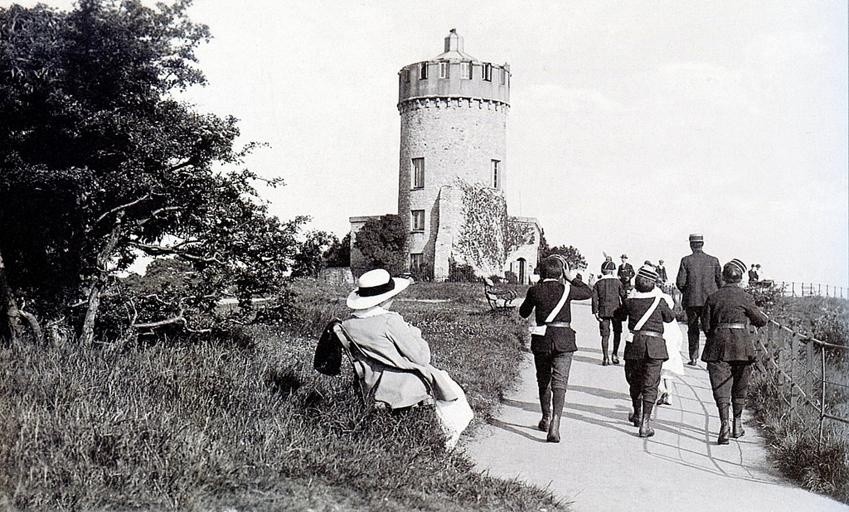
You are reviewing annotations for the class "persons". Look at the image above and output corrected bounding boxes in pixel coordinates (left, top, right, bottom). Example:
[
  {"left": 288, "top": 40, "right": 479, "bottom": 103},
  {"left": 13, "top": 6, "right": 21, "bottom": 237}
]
[
  {"left": 699, "top": 258, "right": 768, "bottom": 446},
  {"left": 343, "top": 268, "right": 475, "bottom": 457},
  {"left": 675, "top": 232, "right": 724, "bottom": 369},
  {"left": 516, "top": 254, "right": 593, "bottom": 442},
  {"left": 590, "top": 254, "right": 676, "bottom": 438},
  {"left": 748, "top": 263, "right": 755, "bottom": 284},
  {"left": 752, "top": 264, "right": 764, "bottom": 283}
]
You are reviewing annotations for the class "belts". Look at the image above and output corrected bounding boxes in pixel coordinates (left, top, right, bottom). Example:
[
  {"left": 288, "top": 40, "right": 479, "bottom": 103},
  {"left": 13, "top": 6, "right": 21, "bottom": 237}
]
[
  {"left": 631, "top": 329, "right": 663, "bottom": 338},
  {"left": 535, "top": 318, "right": 570, "bottom": 329},
  {"left": 712, "top": 321, "right": 746, "bottom": 330}
]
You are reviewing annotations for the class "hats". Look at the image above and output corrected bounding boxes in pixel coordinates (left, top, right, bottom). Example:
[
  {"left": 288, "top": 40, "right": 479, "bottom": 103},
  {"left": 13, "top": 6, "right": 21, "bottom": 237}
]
[
  {"left": 346, "top": 269, "right": 412, "bottom": 309},
  {"left": 689, "top": 233, "right": 704, "bottom": 242},
  {"left": 727, "top": 257, "right": 748, "bottom": 274},
  {"left": 638, "top": 266, "right": 659, "bottom": 281},
  {"left": 621, "top": 254, "right": 628, "bottom": 259}
]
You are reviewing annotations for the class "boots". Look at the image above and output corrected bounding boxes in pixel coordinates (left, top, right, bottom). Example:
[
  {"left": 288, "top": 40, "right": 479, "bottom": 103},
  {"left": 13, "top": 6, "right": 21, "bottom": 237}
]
[
  {"left": 732, "top": 416, "right": 745, "bottom": 438},
  {"left": 547, "top": 414, "right": 560, "bottom": 442},
  {"left": 639, "top": 414, "right": 655, "bottom": 437},
  {"left": 629, "top": 407, "right": 641, "bottom": 426},
  {"left": 718, "top": 419, "right": 730, "bottom": 444},
  {"left": 538, "top": 415, "right": 552, "bottom": 432}
]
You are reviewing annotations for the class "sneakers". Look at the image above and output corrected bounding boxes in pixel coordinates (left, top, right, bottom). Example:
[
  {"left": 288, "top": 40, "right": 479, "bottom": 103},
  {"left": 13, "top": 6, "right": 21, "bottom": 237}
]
[
  {"left": 611, "top": 353, "right": 619, "bottom": 365},
  {"left": 602, "top": 357, "right": 610, "bottom": 366},
  {"left": 688, "top": 358, "right": 697, "bottom": 365}
]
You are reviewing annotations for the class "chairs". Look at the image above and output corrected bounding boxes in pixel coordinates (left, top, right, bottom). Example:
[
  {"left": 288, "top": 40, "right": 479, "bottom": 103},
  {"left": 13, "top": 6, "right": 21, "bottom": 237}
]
[
  {"left": 329, "top": 316, "right": 436, "bottom": 417},
  {"left": 484, "top": 276, "right": 518, "bottom": 310}
]
